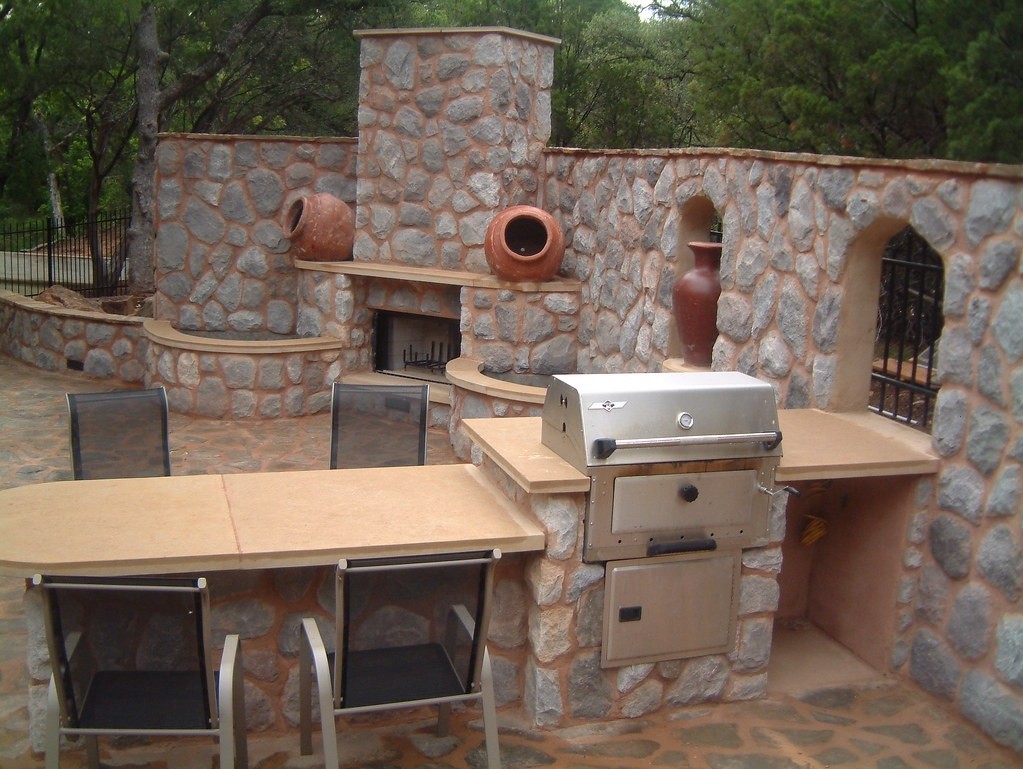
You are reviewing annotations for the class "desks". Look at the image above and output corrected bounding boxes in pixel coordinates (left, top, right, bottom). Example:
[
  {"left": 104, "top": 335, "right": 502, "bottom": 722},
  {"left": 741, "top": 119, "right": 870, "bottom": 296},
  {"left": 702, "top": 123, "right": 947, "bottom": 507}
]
[
  {"left": 461, "top": 419, "right": 938, "bottom": 706},
  {"left": 0, "top": 465, "right": 546, "bottom": 769}
]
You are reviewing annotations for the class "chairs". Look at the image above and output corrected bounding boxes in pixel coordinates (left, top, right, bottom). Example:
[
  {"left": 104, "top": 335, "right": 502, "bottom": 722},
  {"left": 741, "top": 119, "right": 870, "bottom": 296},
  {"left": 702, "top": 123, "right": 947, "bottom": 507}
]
[
  {"left": 31, "top": 573, "right": 249, "bottom": 768},
  {"left": 330, "top": 381, "right": 431, "bottom": 469},
  {"left": 299, "top": 547, "right": 504, "bottom": 769},
  {"left": 66, "top": 386, "right": 171, "bottom": 481}
]
